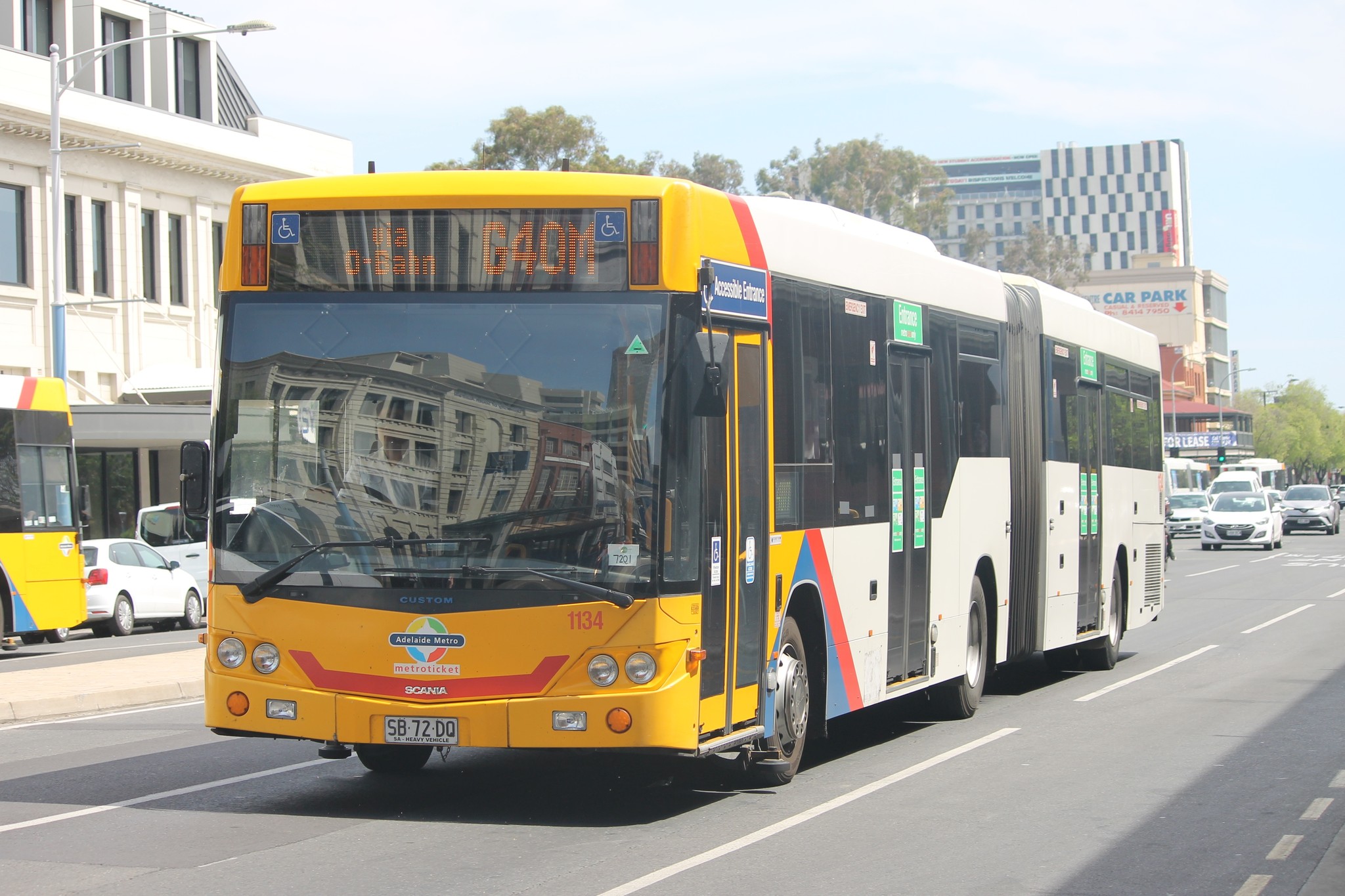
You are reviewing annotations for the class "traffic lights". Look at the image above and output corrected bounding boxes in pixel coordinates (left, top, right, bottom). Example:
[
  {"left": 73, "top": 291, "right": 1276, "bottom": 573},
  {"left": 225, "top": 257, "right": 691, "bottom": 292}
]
[{"left": 1217, "top": 447, "right": 1225, "bottom": 463}]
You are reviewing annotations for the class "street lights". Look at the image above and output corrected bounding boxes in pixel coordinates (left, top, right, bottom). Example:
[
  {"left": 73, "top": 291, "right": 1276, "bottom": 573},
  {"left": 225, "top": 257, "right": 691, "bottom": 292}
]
[
  {"left": 1171, "top": 350, "right": 1213, "bottom": 458},
  {"left": 1217, "top": 366, "right": 1256, "bottom": 473},
  {"left": 46, "top": 17, "right": 280, "bottom": 381}
]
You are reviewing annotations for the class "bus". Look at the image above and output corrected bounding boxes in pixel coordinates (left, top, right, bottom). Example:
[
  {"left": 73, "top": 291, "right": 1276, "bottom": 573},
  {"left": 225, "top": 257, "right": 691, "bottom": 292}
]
[
  {"left": 175, "top": 163, "right": 1174, "bottom": 791},
  {"left": 1218, "top": 457, "right": 1289, "bottom": 498},
  {"left": 1163, "top": 456, "right": 1212, "bottom": 498},
  {"left": 0, "top": 371, "right": 94, "bottom": 652}
]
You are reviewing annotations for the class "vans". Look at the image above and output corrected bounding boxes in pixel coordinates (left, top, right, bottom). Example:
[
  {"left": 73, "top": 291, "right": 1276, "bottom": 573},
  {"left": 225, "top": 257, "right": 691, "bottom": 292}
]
[
  {"left": 132, "top": 497, "right": 209, "bottom": 609},
  {"left": 1206, "top": 470, "right": 1264, "bottom": 505}
]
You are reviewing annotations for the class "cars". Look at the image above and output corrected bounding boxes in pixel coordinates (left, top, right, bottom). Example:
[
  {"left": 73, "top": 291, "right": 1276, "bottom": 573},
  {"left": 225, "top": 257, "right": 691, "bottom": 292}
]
[
  {"left": 81, "top": 537, "right": 205, "bottom": 637},
  {"left": 1280, "top": 482, "right": 1341, "bottom": 535},
  {"left": 1198, "top": 490, "right": 1284, "bottom": 551},
  {"left": 214, "top": 497, "right": 383, "bottom": 587},
  {"left": 1330, "top": 483, "right": 1345, "bottom": 509},
  {"left": 1168, "top": 490, "right": 1215, "bottom": 539}
]
